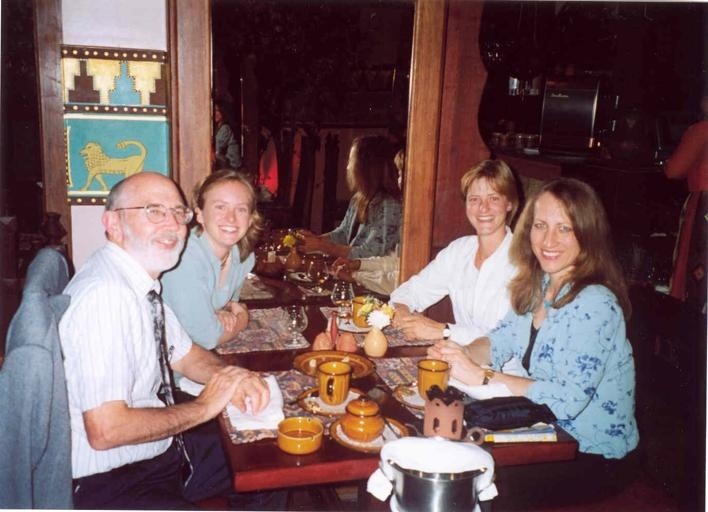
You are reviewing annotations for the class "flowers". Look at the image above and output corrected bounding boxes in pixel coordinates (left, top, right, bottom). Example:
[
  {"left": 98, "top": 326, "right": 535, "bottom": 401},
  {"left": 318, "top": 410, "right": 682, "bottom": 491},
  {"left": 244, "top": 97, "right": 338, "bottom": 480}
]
[
  {"left": 357, "top": 297, "right": 395, "bottom": 329},
  {"left": 283, "top": 228, "right": 305, "bottom": 248}
]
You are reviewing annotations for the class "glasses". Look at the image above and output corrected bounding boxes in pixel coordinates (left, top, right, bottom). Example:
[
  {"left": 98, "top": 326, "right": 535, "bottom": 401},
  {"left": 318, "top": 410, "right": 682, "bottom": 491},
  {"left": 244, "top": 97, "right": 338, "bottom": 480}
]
[{"left": 113, "top": 202, "right": 197, "bottom": 228}]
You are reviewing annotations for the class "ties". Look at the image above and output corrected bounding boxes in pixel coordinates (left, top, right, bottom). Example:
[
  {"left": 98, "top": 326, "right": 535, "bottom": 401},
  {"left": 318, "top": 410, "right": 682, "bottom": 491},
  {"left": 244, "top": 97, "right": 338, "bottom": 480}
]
[{"left": 146, "top": 292, "right": 196, "bottom": 489}]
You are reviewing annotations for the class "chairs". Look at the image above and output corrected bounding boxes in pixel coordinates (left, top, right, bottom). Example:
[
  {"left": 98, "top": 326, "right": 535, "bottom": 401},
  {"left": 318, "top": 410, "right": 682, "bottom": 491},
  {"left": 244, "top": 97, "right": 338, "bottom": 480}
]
[
  {"left": 578, "top": 289, "right": 706, "bottom": 512},
  {"left": 1, "top": 248, "right": 228, "bottom": 510}
]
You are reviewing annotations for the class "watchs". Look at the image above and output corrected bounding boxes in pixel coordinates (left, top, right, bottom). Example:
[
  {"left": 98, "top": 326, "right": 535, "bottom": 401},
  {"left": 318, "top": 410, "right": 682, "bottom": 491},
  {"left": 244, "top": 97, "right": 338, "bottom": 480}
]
[
  {"left": 443, "top": 324, "right": 450, "bottom": 339},
  {"left": 482, "top": 369, "right": 495, "bottom": 384}
]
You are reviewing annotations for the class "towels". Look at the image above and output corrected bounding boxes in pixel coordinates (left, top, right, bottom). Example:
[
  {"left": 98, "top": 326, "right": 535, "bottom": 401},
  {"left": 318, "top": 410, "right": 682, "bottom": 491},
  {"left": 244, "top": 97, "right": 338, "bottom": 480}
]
[
  {"left": 224, "top": 375, "right": 284, "bottom": 430},
  {"left": 450, "top": 368, "right": 512, "bottom": 400}
]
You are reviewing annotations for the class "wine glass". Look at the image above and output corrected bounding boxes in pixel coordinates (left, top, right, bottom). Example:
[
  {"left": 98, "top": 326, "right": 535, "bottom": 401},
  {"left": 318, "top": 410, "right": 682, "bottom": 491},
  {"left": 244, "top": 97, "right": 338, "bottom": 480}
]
[{"left": 278, "top": 258, "right": 354, "bottom": 344}]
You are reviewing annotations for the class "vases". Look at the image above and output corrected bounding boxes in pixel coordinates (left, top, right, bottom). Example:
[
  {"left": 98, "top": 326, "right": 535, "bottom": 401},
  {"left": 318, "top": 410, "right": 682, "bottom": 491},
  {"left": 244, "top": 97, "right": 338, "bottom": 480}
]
[
  {"left": 286, "top": 248, "right": 301, "bottom": 271},
  {"left": 364, "top": 328, "right": 388, "bottom": 357}
]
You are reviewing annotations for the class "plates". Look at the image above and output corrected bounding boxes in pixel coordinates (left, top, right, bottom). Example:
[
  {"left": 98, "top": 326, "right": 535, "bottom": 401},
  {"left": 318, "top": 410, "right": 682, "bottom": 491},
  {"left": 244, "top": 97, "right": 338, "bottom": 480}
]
[
  {"left": 291, "top": 349, "right": 384, "bottom": 382},
  {"left": 391, "top": 385, "right": 462, "bottom": 407},
  {"left": 328, "top": 417, "right": 408, "bottom": 453},
  {"left": 333, "top": 308, "right": 391, "bottom": 335},
  {"left": 295, "top": 386, "right": 371, "bottom": 415}
]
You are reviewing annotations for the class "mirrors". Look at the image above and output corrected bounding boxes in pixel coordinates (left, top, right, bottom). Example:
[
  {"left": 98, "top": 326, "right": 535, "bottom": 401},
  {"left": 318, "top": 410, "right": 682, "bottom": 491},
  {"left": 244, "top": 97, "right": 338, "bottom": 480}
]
[{"left": 173, "top": 0, "right": 445, "bottom": 297}]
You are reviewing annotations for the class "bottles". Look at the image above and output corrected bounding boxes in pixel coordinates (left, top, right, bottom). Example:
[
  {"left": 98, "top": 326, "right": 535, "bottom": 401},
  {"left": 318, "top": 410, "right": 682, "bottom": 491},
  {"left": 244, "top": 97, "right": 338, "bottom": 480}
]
[{"left": 341, "top": 396, "right": 384, "bottom": 442}]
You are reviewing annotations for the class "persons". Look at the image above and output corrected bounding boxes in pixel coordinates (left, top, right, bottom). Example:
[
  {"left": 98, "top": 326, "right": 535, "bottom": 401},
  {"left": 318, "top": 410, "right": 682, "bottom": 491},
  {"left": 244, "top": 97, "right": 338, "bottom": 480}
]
[
  {"left": 58, "top": 171, "right": 270, "bottom": 512},
  {"left": 292, "top": 133, "right": 405, "bottom": 295},
  {"left": 214, "top": 99, "right": 243, "bottom": 170},
  {"left": 428, "top": 178, "right": 639, "bottom": 511},
  {"left": 387, "top": 158, "right": 519, "bottom": 347},
  {"left": 656, "top": 88, "right": 708, "bottom": 319},
  {"left": 159, "top": 169, "right": 269, "bottom": 388}
]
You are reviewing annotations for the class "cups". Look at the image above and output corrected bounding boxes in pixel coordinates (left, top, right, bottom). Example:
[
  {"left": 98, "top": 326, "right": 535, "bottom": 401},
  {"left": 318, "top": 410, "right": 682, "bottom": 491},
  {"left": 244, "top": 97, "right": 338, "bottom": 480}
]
[
  {"left": 351, "top": 294, "right": 378, "bottom": 329},
  {"left": 320, "top": 362, "right": 351, "bottom": 407},
  {"left": 417, "top": 358, "right": 452, "bottom": 399}
]
